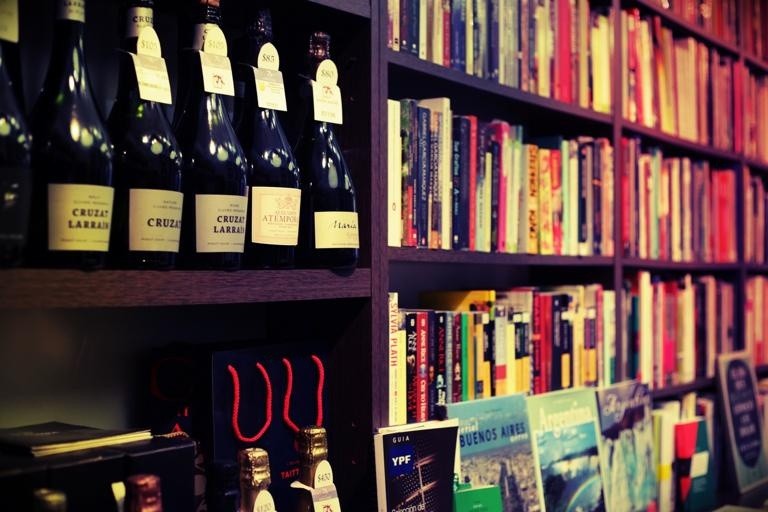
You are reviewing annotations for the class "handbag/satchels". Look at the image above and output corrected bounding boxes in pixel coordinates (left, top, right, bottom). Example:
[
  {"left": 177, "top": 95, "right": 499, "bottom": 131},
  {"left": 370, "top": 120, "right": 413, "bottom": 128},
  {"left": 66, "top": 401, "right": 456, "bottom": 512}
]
[{"left": 170, "top": 344, "right": 330, "bottom": 498}]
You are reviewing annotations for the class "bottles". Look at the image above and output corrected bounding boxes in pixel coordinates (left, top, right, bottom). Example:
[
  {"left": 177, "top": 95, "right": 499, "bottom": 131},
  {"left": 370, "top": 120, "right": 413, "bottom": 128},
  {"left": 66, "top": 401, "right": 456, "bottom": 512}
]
[
  {"left": 294, "top": 30, "right": 360, "bottom": 271},
  {"left": 173, "top": 0, "right": 248, "bottom": 272},
  {"left": 106, "top": 1, "right": 183, "bottom": 272},
  {"left": 232, "top": 9, "right": 302, "bottom": 271},
  {"left": 32, "top": 428, "right": 341, "bottom": 512},
  {"left": 0, "top": 1, "right": 33, "bottom": 267},
  {"left": 32, "top": 1, "right": 115, "bottom": 270}
]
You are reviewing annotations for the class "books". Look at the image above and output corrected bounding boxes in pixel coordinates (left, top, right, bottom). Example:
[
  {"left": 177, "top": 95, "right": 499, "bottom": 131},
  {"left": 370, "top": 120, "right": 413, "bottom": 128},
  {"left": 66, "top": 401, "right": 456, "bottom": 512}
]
[
  {"left": 388, "top": 272, "right": 768, "bottom": 426},
  {"left": 387, "top": 98, "right": 767, "bottom": 264},
  {"left": 0, "top": 421, "right": 154, "bottom": 460},
  {"left": 388, "top": 1, "right": 767, "bottom": 158},
  {"left": 374, "top": 351, "right": 767, "bottom": 511}
]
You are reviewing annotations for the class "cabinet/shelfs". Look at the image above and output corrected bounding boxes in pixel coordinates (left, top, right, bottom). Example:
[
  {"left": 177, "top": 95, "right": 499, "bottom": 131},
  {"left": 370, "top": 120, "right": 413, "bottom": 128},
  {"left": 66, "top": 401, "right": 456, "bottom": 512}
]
[
  {"left": 0, "top": 1, "right": 381, "bottom": 512},
  {"left": 374, "top": 0, "right": 767, "bottom": 511}
]
